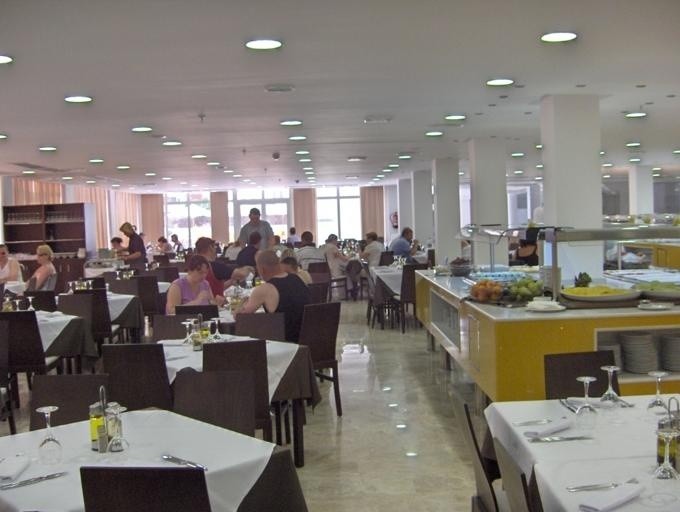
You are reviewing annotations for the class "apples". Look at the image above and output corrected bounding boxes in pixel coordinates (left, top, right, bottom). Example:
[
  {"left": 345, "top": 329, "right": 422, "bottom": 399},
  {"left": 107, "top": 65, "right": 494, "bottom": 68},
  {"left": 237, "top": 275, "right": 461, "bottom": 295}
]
[{"left": 470, "top": 277, "right": 503, "bottom": 302}]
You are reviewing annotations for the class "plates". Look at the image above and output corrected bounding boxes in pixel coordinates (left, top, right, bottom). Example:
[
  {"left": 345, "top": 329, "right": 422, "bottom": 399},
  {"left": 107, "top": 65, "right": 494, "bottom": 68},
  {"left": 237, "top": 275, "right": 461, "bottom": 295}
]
[
  {"left": 638, "top": 302, "right": 673, "bottom": 310},
  {"left": 525, "top": 306, "right": 567, "bottom": 312},
  {"left": 631, "top": 284, "right": 680, "bottom": 298},
  {"left": 597, "top": 333, "right": 680, "bottom": 375},
  {"left": 560, "top": 288, "right": 641, "bottom": 301}
]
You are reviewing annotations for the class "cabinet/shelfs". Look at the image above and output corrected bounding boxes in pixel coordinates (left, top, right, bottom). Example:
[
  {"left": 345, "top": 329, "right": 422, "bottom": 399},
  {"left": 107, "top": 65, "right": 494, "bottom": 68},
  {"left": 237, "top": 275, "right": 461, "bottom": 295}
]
[{"left": 3, "top": 203, "right": 86, "bottom": 253}]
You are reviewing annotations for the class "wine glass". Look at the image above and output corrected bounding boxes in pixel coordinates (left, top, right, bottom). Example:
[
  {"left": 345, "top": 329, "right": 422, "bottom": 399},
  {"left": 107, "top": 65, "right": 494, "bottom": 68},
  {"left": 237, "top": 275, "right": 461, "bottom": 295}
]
[
  {"left": 35, "top": 405, "right": 64, "bottom": 466},
  {"left": 575, "top": 376, "right": 599, "bottom": 432},
  {"left": 116, "top": 269, "right": 135, "bottom": 281},
  {"left": 646, "top": 371, "right": 669, "bottom": 427},
  {"left": 599, "top": 366, "right": 621, "bottom": 419},
  {"left": 104, "top": 406, "right": 131, "bottom": 465},
  {"left": 393, "top": 254, "right": 406, "bottom": 268},
  {"left": 2, "top": 296, "right": 35, "bottom": 312},
  {"left": 649, "top": 429, "right": 680, "bottom": 503},
  {"left": 68, "top": 279, "right": 94, "bottom": 295},
  {"left": 181, "top": 318, "right": 224, "bottom": 351}
]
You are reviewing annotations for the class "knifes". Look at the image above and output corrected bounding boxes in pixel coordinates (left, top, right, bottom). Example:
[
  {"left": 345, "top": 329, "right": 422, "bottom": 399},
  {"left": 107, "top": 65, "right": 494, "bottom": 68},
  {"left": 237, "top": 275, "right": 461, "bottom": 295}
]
[
  {"left": 527, "top": 436, "right": 595, "bottom": 442},
  {"left": 0, "top": 471, "right": 69, "bottom": 491},
  {"left": 162, "top": 454, "right": 209, "bottom": 472}
]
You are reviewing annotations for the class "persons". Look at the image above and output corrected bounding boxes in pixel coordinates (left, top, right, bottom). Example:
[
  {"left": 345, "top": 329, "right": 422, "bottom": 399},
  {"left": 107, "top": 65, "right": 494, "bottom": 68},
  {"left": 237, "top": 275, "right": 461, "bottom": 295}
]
[
  {"left": 195, "top": 237, "right": 251, "bottom": 308},
  {"left": 231, "top": 249, "right": 311, "bottom": 344},
  {"left": 319, "top": 234, "right": 358, "bottom": 298},
  {"left": 0, "top": 244, "right": 24, "bottom": 281},
  {"left": 164, "top": 254, "right": 226, "bottom": 315},
  {"left": 238, "top": 208, "right": 275, "bottom": 249},
  {"left": 111, "top": 222, "right": 183, "bottom": 273},
  {"left": 25, "top": 244, "right": 56, "bottom": 292},
  {"left": 387, "top": 227, "right": 420, "bottom": 264},
  {"left": 216, "top": 227, "right": 326, "bottom": 285},
  {"left": 357, "top": 232, "right": 386, "bottom": 279},
  {"left": 511, "top": 228, "right": 539, "bottom": 264}
]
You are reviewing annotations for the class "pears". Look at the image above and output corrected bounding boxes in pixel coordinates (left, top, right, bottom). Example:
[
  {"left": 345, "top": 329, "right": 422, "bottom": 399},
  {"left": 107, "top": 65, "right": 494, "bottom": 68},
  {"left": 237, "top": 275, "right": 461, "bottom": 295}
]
[{"left": 502, "top": 275, "right": 545, "bottom": 306}]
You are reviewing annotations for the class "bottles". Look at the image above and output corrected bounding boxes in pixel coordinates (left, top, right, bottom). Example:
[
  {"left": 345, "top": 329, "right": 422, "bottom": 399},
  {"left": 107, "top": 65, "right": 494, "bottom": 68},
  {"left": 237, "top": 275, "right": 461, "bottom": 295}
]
[
  {"left": 656, "top": 417, "right": 680, "bottom": 473},
  {"left": 89, "top": 402, "right": 122, "bottom": 451},
  {"left": 228, "top": 277, "right": 263, "bottom": 314}
]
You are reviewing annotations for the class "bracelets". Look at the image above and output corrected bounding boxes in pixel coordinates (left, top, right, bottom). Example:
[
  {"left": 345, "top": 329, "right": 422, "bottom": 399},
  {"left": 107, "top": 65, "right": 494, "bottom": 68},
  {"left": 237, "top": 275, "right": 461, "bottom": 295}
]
[{"left": 413, "top": 244, "right": 418, "bottom": 248}]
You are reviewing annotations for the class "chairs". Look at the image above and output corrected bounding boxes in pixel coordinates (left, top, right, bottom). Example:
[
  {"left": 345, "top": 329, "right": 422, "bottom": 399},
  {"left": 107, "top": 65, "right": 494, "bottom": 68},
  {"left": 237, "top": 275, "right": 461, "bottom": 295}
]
[
  {"left": 461, "top": 403, "right": 499, "bottom": 512},
  {"left": 80, "top": 467, "right": 212, "bottom": 512},
  {"left": 493, "top": 438, "right": 537, "bottom": 512}
]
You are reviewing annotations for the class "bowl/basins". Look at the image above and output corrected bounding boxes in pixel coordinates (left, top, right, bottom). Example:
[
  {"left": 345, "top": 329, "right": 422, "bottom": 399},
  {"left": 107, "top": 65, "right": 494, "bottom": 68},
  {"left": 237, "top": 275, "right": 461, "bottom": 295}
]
[
  {"left": 449, "top": 265, "right": 471, "bottom": 277},
  {"left": 539, "top": 265, "right": 561, "bottom": 290}
]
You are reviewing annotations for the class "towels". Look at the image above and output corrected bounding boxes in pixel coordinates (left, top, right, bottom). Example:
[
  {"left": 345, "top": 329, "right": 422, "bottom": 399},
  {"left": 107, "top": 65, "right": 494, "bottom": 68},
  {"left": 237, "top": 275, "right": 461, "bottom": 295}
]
[
  {"left": 578, "top": 483, "right": 641, "bottom": 512},
  {"left": 525, "top": 418, "right": 572, "bottom": 439},
  {"left": 0, "top": 454, "right": 29, "bottom": 486}
]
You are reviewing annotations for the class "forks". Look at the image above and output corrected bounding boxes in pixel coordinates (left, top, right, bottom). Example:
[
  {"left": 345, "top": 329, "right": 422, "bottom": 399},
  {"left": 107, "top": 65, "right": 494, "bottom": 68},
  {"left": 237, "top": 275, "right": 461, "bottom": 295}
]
[{"left": 568, "top": 478, "right": 640, "bottom": 493}]
